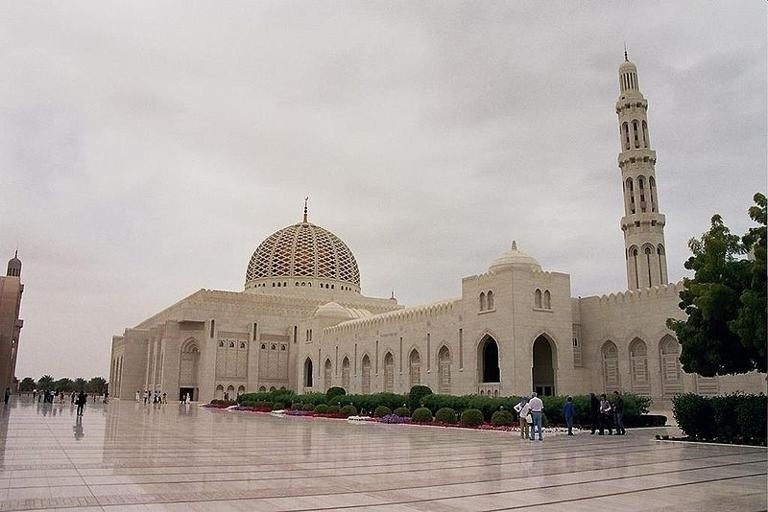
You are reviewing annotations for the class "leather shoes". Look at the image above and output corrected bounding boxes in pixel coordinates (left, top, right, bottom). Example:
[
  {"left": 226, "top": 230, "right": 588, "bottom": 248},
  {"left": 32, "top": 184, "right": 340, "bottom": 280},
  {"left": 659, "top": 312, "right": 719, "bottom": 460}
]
[{"left": 530, "top": 438, "right": 543, "bottom": 440}]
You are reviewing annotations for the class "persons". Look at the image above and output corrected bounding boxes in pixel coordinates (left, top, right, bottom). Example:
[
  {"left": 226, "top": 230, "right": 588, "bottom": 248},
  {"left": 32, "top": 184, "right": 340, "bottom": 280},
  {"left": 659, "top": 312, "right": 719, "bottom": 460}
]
[
  {"left": 590, "top": 391, "right": 626, "bottom": 435},
  {"left": 6, "top": 387, "right": 110, "bottom": 415},
  {"left": 135, "top": 389, "right": 168, "bottom": 404},
  {"left": 224, "top": 392, "right": 240, "bottom": 401},
  {"left": 514, "top": 392, "right": 544, "bottom": 441},
  {"left": 562, "top": 397, "right": 575, "bottom": 435},
  {"left": 181, "top": 392, "right": 190, "bottom": 403}
]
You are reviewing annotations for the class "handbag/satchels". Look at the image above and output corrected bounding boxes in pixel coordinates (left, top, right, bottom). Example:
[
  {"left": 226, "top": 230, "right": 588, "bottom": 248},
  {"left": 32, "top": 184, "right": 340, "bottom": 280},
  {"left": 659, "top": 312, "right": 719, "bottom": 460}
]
[
  {"left": 526, "top": 413, "right": 533, "bottom": 423},
  {"left": 517, "top": 413, "right": 520, "bottom": 418}
]
